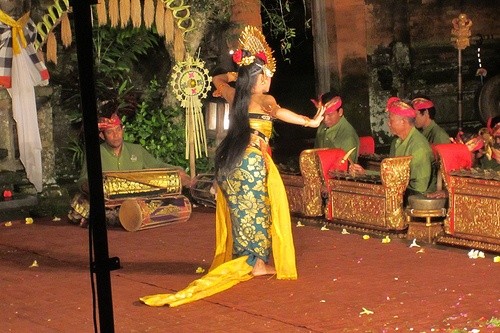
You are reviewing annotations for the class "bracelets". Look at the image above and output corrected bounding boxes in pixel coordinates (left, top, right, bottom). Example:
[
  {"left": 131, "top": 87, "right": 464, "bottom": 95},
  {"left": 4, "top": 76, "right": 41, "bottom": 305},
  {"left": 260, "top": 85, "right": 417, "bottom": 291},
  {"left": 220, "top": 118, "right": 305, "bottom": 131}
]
[
  {"left": 304, "top": 116, "right": 309, "bottom": 126},
  {"left": 228, "top": 71, "right": 237, "bottom": 81}
]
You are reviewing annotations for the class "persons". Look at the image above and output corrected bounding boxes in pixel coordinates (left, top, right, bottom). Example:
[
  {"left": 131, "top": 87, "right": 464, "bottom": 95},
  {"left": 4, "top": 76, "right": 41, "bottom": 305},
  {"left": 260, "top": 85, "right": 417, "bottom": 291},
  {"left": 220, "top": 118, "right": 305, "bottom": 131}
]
[
  {"left": 76, "top": 113, "right": 190, "bottom": 186},
  {"left": 311, "top": 91, "right": 359, "bottom": 164},
  {"left": 139, "top": 56, "right": 325, "bottom": 307},
  {"left": 349, "top": 97, "right": 431, "bottom": 205},
  {"left": 412, "top": 98, "right": 450, "bottom": 192},
  {"left": 427, "top": 124, "right": 500, "bottom": 198}
]
